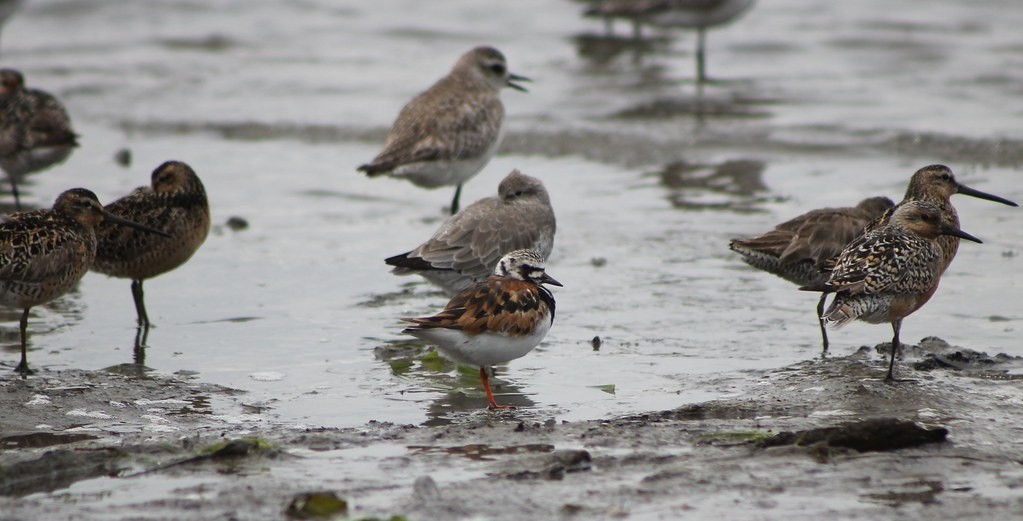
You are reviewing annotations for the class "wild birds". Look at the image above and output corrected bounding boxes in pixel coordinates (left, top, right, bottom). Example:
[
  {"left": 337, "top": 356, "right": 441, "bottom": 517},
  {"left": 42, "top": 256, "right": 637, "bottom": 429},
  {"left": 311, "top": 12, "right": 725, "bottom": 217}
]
[
  {"left": 729, "top": 165, "right": 1018, "bottom": 386},
  {"left": 1, "top": 67, "right": 81, "bottom": 210},
  {"left": 86, "top": 162, "right": 209, "bottom": 321},
  {"left": 579, "top": 0, "right": 761, "bottom": 82},
  {"left": 0, "top": 189, "right": 175, "bottom": 378},
  {"left": 383, "top": 171, "right": 555, "bottom": 300},
  {"left": 357, "top": 44, "right": 532, "bottom": 217},
  {"left": 398, "top": 246, "right": 564, "bottom": 411}
]
